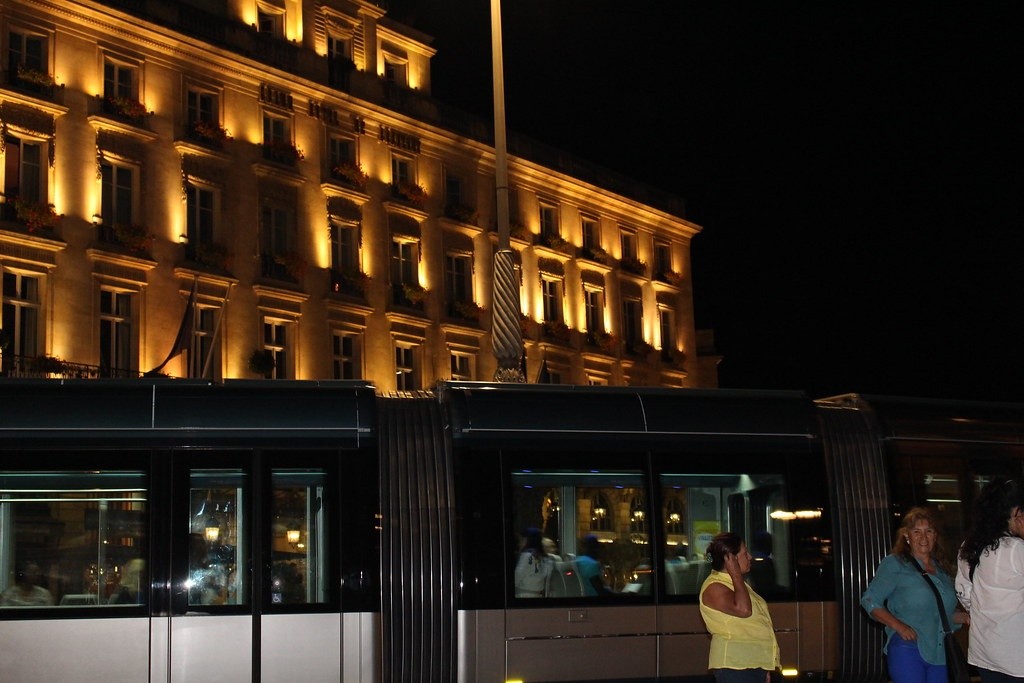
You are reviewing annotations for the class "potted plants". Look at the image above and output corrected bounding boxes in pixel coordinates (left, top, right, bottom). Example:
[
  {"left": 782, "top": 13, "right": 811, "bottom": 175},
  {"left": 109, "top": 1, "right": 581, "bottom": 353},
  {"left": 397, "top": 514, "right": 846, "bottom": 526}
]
[{"left": 0, "top": 64, "right": 687, "bottom": 379}]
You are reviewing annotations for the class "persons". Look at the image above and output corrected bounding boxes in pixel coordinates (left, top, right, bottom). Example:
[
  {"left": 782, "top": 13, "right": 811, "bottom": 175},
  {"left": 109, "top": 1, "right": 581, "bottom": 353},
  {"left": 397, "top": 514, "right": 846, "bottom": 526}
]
[
  {"left": 0, "top": 559, "right": 140, "bottom": 606},
  {"left": 189, "top": 533, "right": 236, "bottom": 606},
  {"left": 861, "top": 507, "right": 970, "bottom": 683},
  {"left": 699, "top": 533, "right": 782, "bottom": 683},
  {"left": 514, "top": 528, "right": 641, "bottom": 600},
  {"left": 954, "top": 478, "right": 1024, "bottom": 683}
]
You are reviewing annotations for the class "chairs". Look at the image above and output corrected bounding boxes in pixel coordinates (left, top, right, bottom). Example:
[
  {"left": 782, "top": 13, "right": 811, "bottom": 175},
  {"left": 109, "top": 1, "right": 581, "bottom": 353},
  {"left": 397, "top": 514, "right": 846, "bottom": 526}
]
[
  {"left": 563, "top": 552, "right": 586, "bottom": 598},
  {"left": 664, "top": 554, "right": 706, "bottom": 597},
  {"left": 545, "top": 554, "right": 573, "bottom": 598}
]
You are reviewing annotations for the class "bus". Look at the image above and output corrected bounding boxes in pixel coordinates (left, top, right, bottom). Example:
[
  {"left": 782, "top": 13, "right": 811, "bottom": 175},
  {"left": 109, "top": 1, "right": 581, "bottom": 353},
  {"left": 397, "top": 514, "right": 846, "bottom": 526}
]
[{"left": 0, "top": 377, "right": 1022, "bottom": 683}]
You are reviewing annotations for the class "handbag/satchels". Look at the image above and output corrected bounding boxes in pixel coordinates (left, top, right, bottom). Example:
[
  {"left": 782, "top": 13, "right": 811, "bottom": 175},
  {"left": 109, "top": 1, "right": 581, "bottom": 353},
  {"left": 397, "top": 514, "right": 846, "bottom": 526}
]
[{"left": 945, "top": 633, "right": 969, "bottom": 683}]
